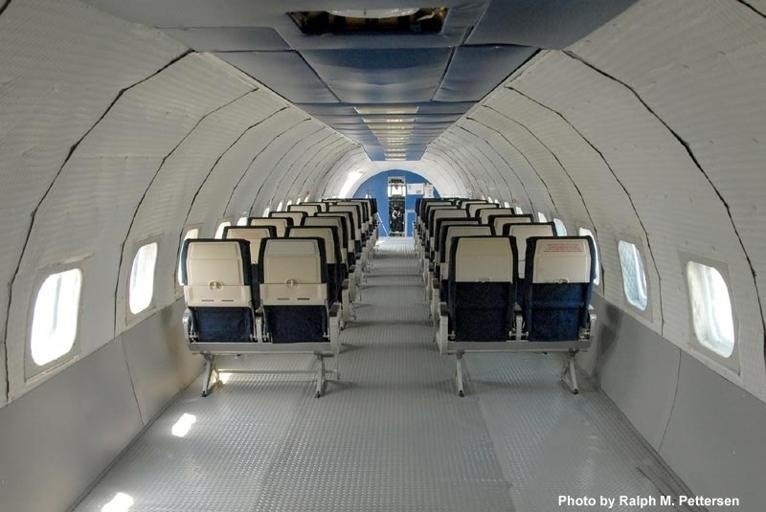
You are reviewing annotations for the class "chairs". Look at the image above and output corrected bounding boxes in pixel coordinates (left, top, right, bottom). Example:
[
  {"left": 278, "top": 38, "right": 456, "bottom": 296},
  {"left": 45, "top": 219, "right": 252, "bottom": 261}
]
[{"left": 415, "top": 197, "right": 598, "bottom": 397}]
